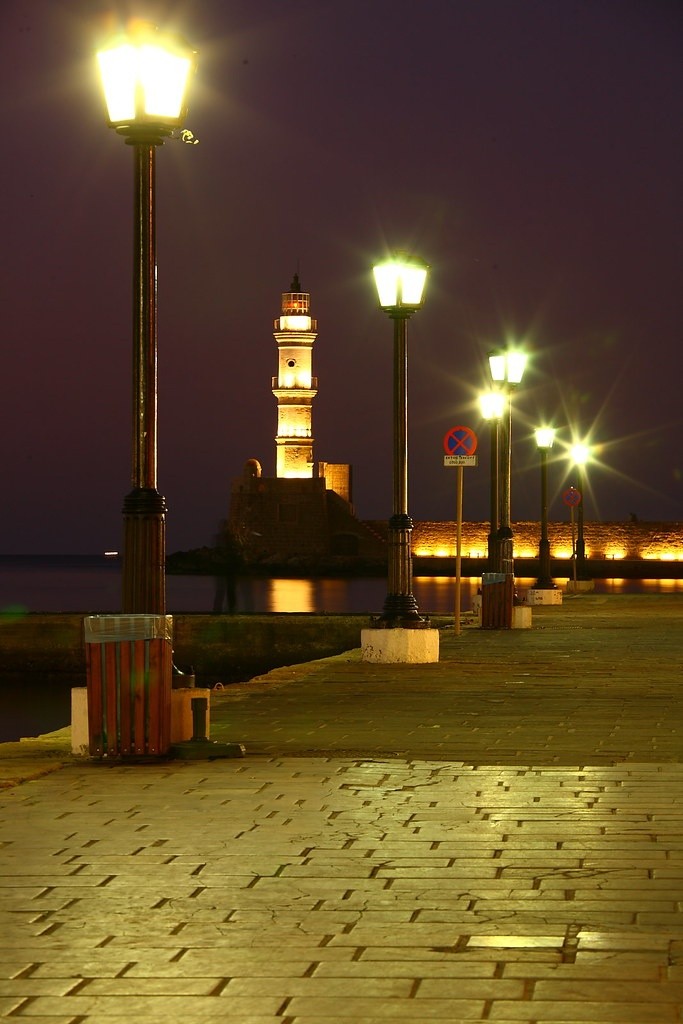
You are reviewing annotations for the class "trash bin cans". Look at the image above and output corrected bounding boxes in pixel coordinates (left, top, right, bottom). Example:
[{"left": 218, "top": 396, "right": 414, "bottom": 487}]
[
  {"left": 85, "top": 614, "right": 174, "bottom": 758},
  {"left": 482, "top": 572, "right": 514, "bottom": 629}
]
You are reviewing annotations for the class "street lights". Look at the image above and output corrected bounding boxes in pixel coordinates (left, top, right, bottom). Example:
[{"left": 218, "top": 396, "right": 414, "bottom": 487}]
[
  {"left": 566, "top": 437, "right": 595, "bottom": 581},
  {"left": 477, "top": 387, "right": 508, "bottom": 573},
  {"left": 485, "top": 333, "right": 531, "bottom": 607},
  {"left": 531, "top": 421, "right": 560, "bottom": 591},
  {"left": 367, "top": 246, "right": 433, "bottom": 630},
  {"left": 88, "top": 20, "right": 204, "bottom": 616}
]
